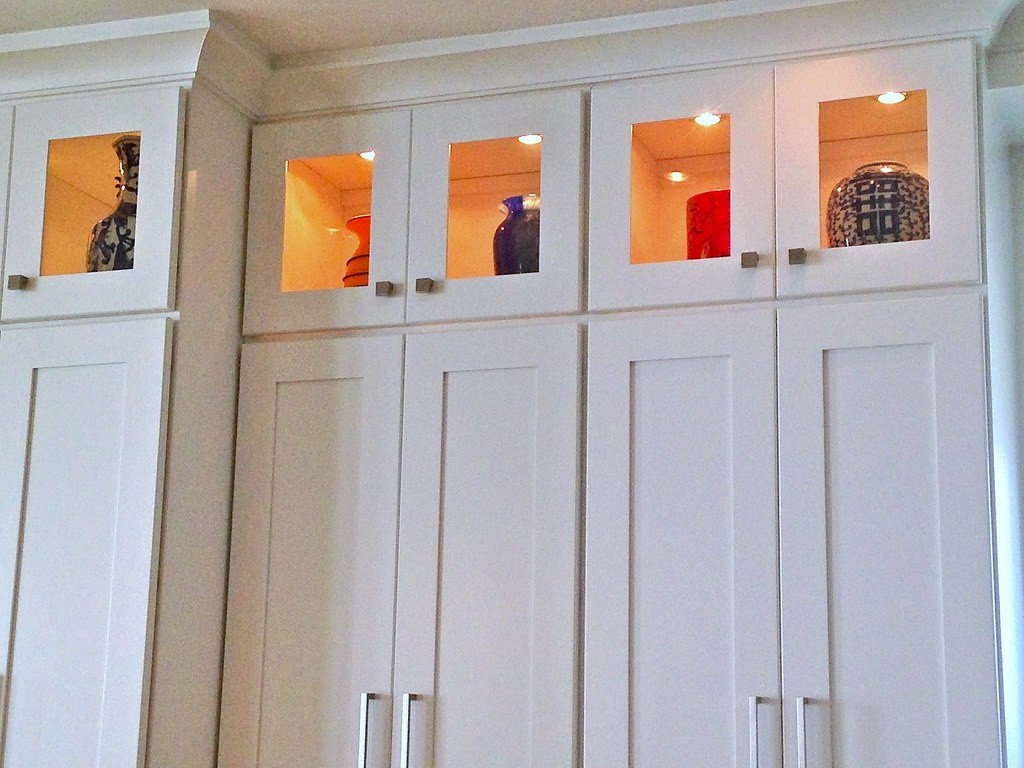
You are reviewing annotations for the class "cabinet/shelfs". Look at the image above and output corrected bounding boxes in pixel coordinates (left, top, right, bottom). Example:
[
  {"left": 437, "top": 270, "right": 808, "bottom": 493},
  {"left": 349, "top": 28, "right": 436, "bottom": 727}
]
[{"left": 2, "top": 1, "right": 1022, "bottom": 768}]
